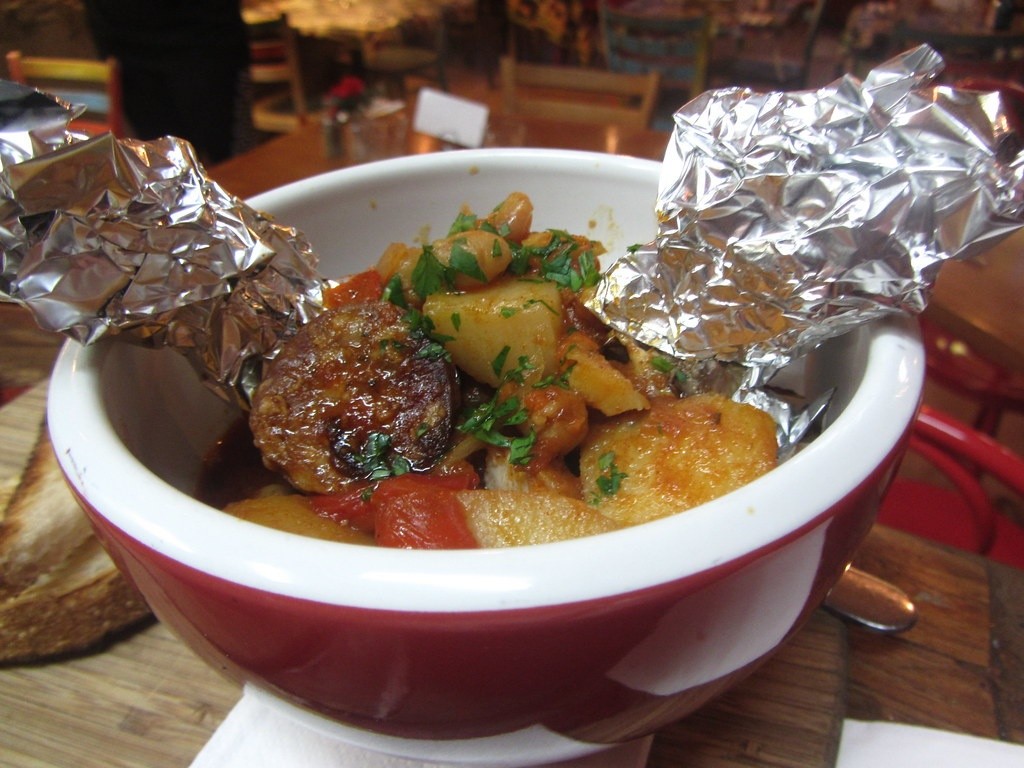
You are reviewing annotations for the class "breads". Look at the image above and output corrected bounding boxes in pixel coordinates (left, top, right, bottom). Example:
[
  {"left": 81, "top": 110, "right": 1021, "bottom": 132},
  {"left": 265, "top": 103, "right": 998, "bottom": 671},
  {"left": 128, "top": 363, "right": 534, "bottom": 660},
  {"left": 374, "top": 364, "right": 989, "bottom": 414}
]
[{"left": 0, "top": 407, "right": 151, "bottom": 665}]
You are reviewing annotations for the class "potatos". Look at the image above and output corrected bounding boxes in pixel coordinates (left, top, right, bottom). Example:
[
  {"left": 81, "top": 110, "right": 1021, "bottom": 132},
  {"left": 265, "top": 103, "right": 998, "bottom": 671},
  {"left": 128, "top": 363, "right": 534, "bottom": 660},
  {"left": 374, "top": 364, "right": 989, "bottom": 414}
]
[
  {"left": 222, "top": 494, "right": 375, "bottom": 544},
  {"left": 426, "top": 232, "right": 780, "bottom": 542}
]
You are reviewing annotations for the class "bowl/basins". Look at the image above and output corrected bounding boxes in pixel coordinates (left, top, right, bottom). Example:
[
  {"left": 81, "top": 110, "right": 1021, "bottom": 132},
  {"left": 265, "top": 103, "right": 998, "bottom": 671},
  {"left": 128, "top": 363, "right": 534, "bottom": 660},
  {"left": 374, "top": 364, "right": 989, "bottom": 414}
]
[{"left": 45, "top": 144, "right": 924, "bottom": 768}]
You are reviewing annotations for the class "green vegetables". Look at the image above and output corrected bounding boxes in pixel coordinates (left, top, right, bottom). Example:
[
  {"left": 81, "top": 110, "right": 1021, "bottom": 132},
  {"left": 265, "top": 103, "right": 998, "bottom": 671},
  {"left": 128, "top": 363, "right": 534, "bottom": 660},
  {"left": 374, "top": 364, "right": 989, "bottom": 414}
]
[{"left": 370, "top": 212, "right": 682, "bottom": 493}]
